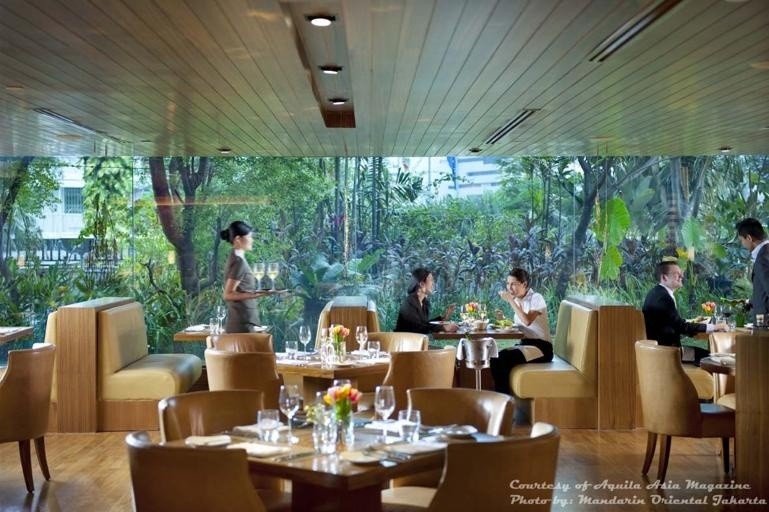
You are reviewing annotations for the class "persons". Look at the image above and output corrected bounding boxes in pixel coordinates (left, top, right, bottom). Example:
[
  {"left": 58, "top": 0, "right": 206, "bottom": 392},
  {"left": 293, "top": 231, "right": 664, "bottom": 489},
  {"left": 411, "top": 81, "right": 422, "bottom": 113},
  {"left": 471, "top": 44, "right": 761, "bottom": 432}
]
[
  {"left": 640, "top": 261, "right": 731, "bottom": 404},
  {"left": 734, "top": 216, "right": 769, "bottom": 328},
  {"left": 486, "top": 268, "right": 555, "bottom": 427},
  {"left": 217, "top": 220, "right": 272, "bottom": 335},
  {"left": 394, "top": 268, "right": 460, "bottom": 350}
]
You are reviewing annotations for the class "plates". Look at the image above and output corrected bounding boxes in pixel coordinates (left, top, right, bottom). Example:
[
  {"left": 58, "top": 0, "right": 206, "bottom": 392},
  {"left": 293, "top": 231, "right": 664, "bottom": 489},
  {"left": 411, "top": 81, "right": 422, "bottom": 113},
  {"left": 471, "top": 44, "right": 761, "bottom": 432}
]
[
  {"left": 495, "top": 328, "right": 519, "bottom": 334},
  {"left": 184, "top": 433, "right": 232, "bottom": 450},
  {"left": 184, "top": 326, "right": 204, "bottom": 332},
  {"left": 431, "top": 424, "right": 477, "bottom": 438},
  {"left": 253, "top": 326, "right": 267, "bottom": 332},
  {"left": 490, "top": 323, "right": 518, "bottom": 329},
  {"left": 342, "top": 449, "right": 386, "bottom": 466}
]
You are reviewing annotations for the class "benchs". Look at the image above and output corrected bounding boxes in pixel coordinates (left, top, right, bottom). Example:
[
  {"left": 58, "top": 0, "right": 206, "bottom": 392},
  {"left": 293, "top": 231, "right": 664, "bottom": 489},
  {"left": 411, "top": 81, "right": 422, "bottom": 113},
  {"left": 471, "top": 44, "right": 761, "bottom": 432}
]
[
  {"left": 276, "top": 296, "right": 380, "bottom": 357},
  {"left": 510, "top": 295, "right": 715, "bottom": 430},
  {"left": 0, "top": 296, "right": 203, "bottom": 431}
]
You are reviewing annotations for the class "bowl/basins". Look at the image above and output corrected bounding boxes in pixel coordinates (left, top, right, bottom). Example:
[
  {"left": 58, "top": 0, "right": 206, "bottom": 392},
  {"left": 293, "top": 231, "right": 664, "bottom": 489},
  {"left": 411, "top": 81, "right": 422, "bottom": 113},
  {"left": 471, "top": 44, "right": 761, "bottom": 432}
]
[{"left": 475, "top": 321, "right": 488, "bottom": 330}]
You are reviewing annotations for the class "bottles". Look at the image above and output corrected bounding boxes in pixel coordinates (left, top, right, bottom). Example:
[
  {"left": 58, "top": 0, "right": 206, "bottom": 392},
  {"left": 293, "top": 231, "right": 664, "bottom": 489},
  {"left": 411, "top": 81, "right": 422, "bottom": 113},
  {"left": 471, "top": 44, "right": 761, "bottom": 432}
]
[{"left": 719, "top": 297, "right": 750, "bottom": 315}]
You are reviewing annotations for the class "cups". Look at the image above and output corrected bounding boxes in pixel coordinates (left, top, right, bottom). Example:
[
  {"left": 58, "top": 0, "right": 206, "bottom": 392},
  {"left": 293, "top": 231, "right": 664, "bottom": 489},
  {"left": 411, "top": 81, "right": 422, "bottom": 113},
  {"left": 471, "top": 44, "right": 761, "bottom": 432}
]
[
  {"left": 368, "top": 340, "right": 381, "bottom": 360},
  {"left": 285, "top": 341, "right": 299, "bottom": 360},
  {"left": 311, "top": 423, "right": 337, "bottom": 453},
  {"left": 207, "top": 318, "right": 219, "bottom": 332},
  {"left": 255, "top": 409, "right": 279, "bottom": 445},
  {"left": 397, "top": 410, "right": 420, "bottom": 443}
]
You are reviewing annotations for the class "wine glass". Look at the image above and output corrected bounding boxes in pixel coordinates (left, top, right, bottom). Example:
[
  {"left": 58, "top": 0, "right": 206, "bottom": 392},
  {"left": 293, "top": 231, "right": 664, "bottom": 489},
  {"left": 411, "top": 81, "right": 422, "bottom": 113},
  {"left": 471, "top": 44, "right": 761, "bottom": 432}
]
[
  {"left": 477, "top": 303, "right": 487, "bottom": 323},
  {"left": 714, "top": 305, "right": 737, "bottom": 331},
  {"left": 459, "top": 305, "right": 467, "bottom": 328},
  {"left": 252, "top": 262, "right": 264, "bottom": 292},
  {"left": 279, "top": 379, "right": 397, "bottom": 445},
  {"left": 299, "top": 322, "right": 367, "bottom": 370},
  {"left": 215, "top": 305, "right": 226, "bottom": 332},
  {"left": 266, "top": 263, "right": 280, "bottom": 290}
]
